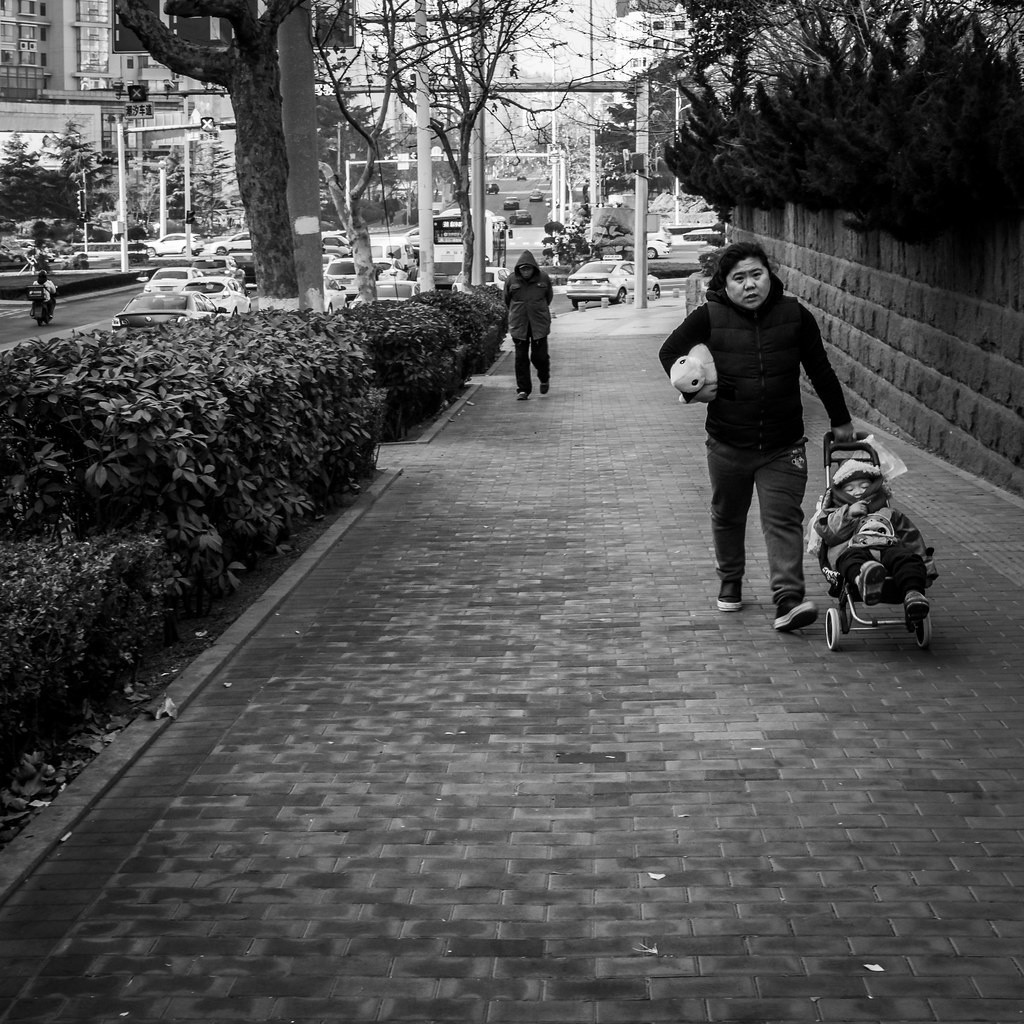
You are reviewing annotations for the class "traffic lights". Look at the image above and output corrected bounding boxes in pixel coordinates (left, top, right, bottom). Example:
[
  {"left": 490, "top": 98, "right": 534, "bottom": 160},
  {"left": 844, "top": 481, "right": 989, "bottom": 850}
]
[
  {"left": 97, "top": 154, "right": 118, "bottom": 165},
  {"left": 186, "top": 210, "right": 195, "bottom": 224},
  {"left": 76, "top": 191, "right": 85, "bottom": 213},
  {"left": 127, "top": 85, "right": 148, "bottom": 104}
]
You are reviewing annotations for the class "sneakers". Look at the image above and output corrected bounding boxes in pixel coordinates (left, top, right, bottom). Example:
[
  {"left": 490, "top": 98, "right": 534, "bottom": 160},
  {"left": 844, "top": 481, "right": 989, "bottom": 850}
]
[
  {"left": 717, "top": 578, "right": 744, "bottom": 611},
  {"left": 774, "top": 598, "right": 817, "bottom": 630}
]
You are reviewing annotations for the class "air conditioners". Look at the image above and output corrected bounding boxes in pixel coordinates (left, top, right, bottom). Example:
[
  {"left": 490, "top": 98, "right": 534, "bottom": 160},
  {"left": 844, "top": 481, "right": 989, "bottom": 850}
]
[
  {"left": 20, "top": 42, "right": 28, "bottom": 50},
  {"left": 29, "top": 43, "right": 38, "bottom": 50}
]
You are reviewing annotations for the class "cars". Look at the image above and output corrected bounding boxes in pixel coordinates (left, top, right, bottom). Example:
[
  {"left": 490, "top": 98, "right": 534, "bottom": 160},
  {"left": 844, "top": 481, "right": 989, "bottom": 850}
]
[
  {"left": 227, "top": 253, "right": 257, "bottom": 283},
  {"left": 316, "top": 231, "right": 420, "bottom": 310},
  {"left": 144, "top": 266, "right": 205, "bottom": 292},
  {"left": 178, "top": 277, "right": 253, "bottom": 317},
  {"left": 143, "top": 233, "right": 206, "bottom": 256},
  {"left": 614, "top": 224, "right": 673, "bottom": 260},
  {"left": 194, "top": 255, "right": 248, "bottom": 286},
  {"left": 0, "top": 238, "right": 57, "bottom": 266},
  {"left": 209, "top": 231, "right": 252, "bottom": 256},
  {"left": 483, "top": 171, "right": 546, "bottom": 225},
  {"left": 565, "top": 260, "right": 661, "bottom": 309},
  {"left": 111, "top": 291, "right": 228, "bottom": 332}
]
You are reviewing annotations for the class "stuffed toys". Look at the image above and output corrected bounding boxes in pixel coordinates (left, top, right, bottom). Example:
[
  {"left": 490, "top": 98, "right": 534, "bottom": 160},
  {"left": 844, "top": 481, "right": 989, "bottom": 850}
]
[{"left": 670, "top": 343, "right": 717, "bottom": 404}]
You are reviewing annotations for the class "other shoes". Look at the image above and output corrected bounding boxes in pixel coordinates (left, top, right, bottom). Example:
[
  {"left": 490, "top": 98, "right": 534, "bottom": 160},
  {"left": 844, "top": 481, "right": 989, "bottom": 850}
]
[
  {"left": 517, "top": 392, "right": 528, "bottom": 400},
  {"left": 857, "top": 561, "right": 885, "bottom": 605},
  {"left": 906, "top": 592, "right": 930, "bottom": 621},
  {"left": 540, "top": 378, "right": 549, "bottom": 394}
]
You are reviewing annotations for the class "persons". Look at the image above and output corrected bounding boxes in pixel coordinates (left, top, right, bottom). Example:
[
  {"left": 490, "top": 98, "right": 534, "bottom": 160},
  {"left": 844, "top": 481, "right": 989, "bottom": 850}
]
[
  {"left": 504, "top": 250, "right": 553, "bottom": 399},
  {"left": 34, "top": 274, "right": 56, "bottom": 318},
  {"left": 659, "top": 240, "right": 856, "bottom": 633},
  {"left": 813, "top": 460, "right": 939, "bottom": 628}
]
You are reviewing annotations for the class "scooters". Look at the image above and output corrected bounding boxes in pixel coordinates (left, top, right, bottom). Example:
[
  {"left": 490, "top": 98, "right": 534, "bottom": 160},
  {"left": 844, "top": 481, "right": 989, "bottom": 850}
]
[{"left": 24, "top": 285, "right": 59, "bottom": 328}]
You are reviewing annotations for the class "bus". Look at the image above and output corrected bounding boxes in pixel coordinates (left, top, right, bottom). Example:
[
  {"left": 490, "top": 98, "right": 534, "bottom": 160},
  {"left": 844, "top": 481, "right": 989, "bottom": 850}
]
[{"left": 427, "top": 208, "right": 514, "bottom": 287}]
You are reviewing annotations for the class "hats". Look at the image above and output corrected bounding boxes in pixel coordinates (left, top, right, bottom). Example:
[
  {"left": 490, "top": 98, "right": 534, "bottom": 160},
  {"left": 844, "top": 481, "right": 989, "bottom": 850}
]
[
  {"left": 834, "top": 457, "right": 881, "bottom": 483},
  {"left": 519, "top": 265, "right": 532, "bottom": 268}
]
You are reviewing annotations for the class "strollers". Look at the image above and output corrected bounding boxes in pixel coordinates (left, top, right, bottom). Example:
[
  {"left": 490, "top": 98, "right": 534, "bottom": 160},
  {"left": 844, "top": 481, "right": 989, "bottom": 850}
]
[{"left": 822, "top": 430, "right": 935, "bottom": 652}]
